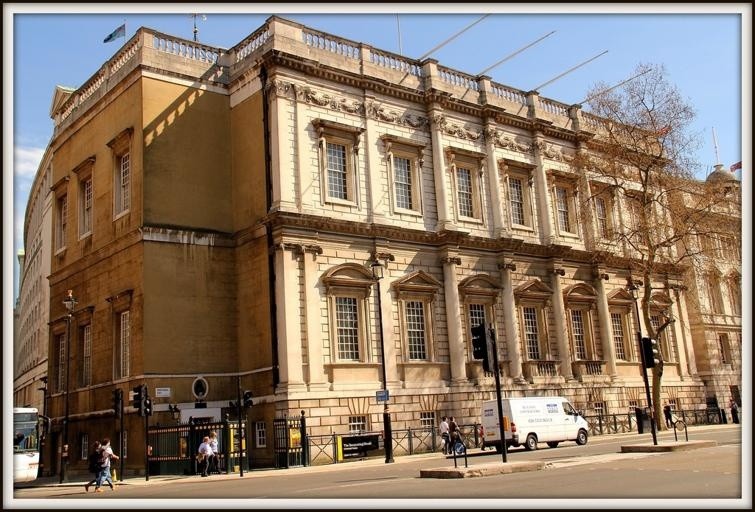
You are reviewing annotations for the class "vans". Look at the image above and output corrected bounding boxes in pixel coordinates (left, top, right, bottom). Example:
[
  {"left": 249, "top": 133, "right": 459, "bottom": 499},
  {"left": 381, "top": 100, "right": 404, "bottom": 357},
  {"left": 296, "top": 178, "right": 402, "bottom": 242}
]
[{"left": 481, "top": 397, "right": 589, "bottom": 453}]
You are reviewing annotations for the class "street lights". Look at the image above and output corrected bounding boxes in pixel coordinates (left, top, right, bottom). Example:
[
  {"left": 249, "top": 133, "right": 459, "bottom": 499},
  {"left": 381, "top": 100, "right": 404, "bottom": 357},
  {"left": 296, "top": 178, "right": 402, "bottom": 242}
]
[
  {"left": 60, "top": 288, "right": 79, "bottom": 484},
  {"left": 371, "top": 260, "right": 395, "bottom": 464},
  {"left": 37, "top": 375, "right": 48, "bottom": 478},
  {"left": 628, "top": 284, "right": 658, "bottom": 445}
]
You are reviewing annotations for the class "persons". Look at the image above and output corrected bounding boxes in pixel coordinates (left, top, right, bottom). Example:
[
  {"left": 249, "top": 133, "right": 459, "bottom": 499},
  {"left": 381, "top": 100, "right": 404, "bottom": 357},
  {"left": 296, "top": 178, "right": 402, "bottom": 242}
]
[
  {"left": 480, "top": 425, "right": 495, "bottom": 451},
  {"left": 85, "top": 440, "right": 103, "bottom": 492},
  {"left": 207, "top": 431, "right": 222, "bottom": 475},
  {"left": 94, "top": 438, "right": 120, "bottom": 492},
  {"left": 449, "top": 416, "right": 461, "bottom": 454},
  {"left": 197, "top": 436, "right": 214, "bottom": 476},
  {"left": 729, "top": 398, "right": 739, "bottom": 423},
  {"left": 61, "top": 443, "right": 70, "bottom": 483},
  {"left": 440, "top": 416, "right": 450, "bottom": 455},
  {"left": 663, "top": 400, "right": 673, "bottom": 427}
]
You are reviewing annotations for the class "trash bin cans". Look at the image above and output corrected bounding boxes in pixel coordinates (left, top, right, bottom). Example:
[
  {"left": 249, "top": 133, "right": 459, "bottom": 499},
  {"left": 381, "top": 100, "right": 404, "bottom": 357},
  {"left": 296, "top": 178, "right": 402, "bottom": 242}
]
[{"left": 636, "top": 407, "right": 652, "bottom": 433}]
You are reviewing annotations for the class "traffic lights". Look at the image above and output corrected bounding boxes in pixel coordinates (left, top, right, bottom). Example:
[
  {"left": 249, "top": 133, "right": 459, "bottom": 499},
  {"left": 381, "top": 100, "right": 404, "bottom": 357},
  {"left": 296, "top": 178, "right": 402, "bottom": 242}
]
[
  {"left": 642, "top": 337, "right": 659, "bottom": 368},
  {"left": 471, "top": 321, "right": 494, "bottom": 372},
  {"left": 132, "top": 384, "right": 144, "bottom": 417},
  {"left": 145, "top": 398, "right": 153, "bottom": 416},
  {"left": 244, "top": 388, "right": 253, "bottom": 409},
  {"left": 112, "top": 388, "right": 122, "bottom": 420}
]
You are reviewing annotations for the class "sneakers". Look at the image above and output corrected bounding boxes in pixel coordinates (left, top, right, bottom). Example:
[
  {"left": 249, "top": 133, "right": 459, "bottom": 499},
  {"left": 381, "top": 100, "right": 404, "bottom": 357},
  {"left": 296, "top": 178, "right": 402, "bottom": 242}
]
[
  {"left": 95, "top": 487, "right": 104, "bottom": 492},
  {"left": 85, "top": 484, "right": 89, "bottom": 492},
  {"left": 110, "top": 485, "right": 115, "bottom": 491}
]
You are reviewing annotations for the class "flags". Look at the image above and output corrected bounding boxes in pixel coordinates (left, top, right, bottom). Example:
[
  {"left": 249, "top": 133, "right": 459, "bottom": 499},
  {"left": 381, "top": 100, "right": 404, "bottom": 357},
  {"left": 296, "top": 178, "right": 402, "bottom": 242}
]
[
  {"left": 729, "top": 160, "right": 742, "bottom": 173},
  {"left": 103, "top": 23, "right": 126, "bottom": 44}
]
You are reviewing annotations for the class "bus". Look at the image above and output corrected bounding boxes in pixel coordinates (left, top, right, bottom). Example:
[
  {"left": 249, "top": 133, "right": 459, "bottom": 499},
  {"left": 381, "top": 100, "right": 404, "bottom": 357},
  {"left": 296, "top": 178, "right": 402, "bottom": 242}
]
[{"left": 13, "top": 407, "right": 52, "bottom": 483}]
